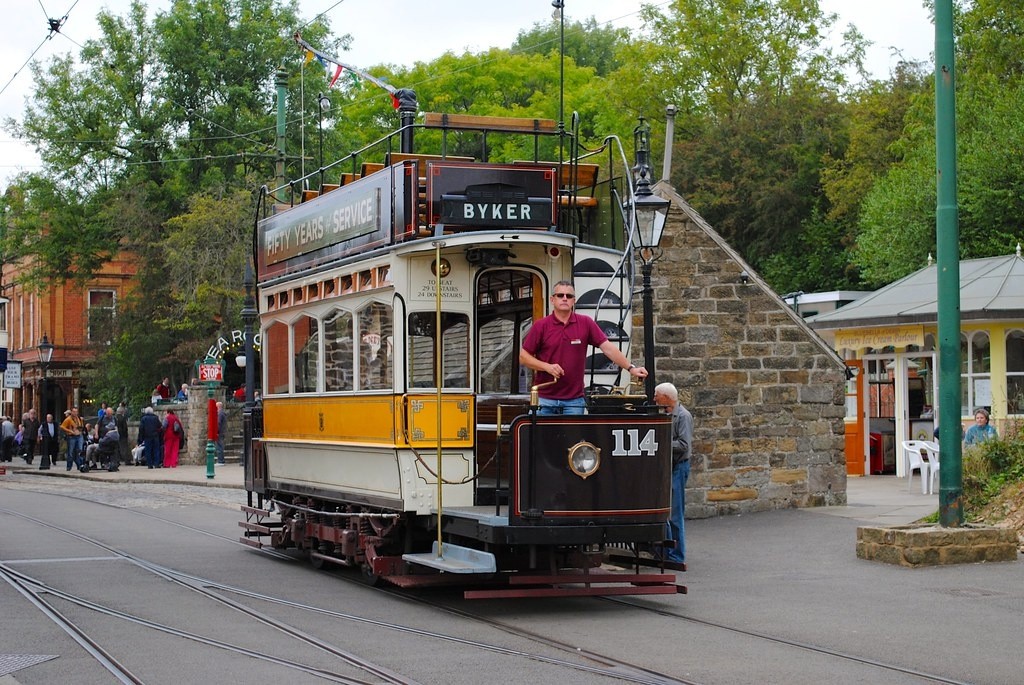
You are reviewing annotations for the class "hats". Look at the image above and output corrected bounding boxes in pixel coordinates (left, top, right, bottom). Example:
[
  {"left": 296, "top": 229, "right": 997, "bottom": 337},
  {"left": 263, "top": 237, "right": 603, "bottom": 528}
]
[
  {"left": 64, "top": 410, "right": 71, "bottom": 414},
  {"left": 105, "top": 422, "right": 116, "bottom": 429}
]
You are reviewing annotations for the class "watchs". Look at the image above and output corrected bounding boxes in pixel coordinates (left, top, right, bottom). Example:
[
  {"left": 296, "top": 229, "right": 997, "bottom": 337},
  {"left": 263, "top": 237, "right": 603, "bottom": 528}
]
[{"left": 628, "top": 365, "right": 635, "bottom": 372}]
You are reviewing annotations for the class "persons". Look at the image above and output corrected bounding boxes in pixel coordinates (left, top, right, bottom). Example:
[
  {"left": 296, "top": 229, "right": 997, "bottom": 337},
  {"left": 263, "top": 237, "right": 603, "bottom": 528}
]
[
  {"left": 137, "top": 407, "right": 162, "bottom": 468},
  {"left": 60, "top": 407, "right": 84, "bottom": 471},
  {"left": 64, "top": 410, "right": 71, "bottom": 417},
  {"left": 151, "top": 389, "right": 162, "bottom": 403},
  {"left": 156, "top": 377, "right": 169, "bottom": 398},
  {"left": 0, "top": 415, "right": 16, "bottom": 462},
  {"left": 161, "top": 409, "right": 183, "bottom": 468},
  {"left": 85, "top": 402, "right": 134, "bottom": 468},
  {"left": 38, "top": 414, "right": 59, "bottom": 465},
  {"left": 214, "top": 402, "right": 226, "bottom": 466},
  {"left": 653, "top": 383, "right": 693, "bottom": 562},
  {"left": 933, "top": 409, "right": 998, "bottom": 449},
  {"left": 233, "top": 383, "right": 246, "bottom": 402},
  {"left": 178, "top": 383, "right": 188, "bottom": 401},
  {"left": 18, "top": 409, "right": 40, "bottom": 459},
  {"left": 518, "top": 281, "right": 648, "bottom": 415}
]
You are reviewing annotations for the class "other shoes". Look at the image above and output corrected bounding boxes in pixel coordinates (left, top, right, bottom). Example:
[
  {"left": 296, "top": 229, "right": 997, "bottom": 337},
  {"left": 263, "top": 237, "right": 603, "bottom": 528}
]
[
  {"left": 155, "top": 466, "right": 161, "bottom": 468},
  {"left": 90, "top": 464, "right": 97, "bottom": 468},
  {"left": 136, "top": 462, "right": 139, "bottom": 466},
  {"left": 125, "top": 463, "right": 134, "bottom": 466},
  {"left": 67, "top": 468, "right": 71, "bottom": 471},
  {"left": 142, "top": 461, "right": 144, "bottom": 464},
  {"left": 214, "top": 462, "right": 225, "bottom": 467},
  {"left": 148, "top": 466, "right": 152, "bottom": 469},
  {"left": 53, "top": 462, "right": 56, "bottom": 465},
  {"left": 85, "top": 461, "right": 89, "bottom": 465}
]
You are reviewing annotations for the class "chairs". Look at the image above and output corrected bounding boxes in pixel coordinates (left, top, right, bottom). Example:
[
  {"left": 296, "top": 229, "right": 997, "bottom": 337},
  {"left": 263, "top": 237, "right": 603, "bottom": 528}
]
[
  {"left": 921, "top": 441, "right": 939, "bottom": 494},
  {"left": 901, "top": 439, "right": 930, "bottom": 494}
]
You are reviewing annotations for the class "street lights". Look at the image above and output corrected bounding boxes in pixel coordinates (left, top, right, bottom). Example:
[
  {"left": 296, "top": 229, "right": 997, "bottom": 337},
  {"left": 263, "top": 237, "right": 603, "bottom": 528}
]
[
  {"left": 612, "top": 168, "right": 672, "bottom": 563},
  {"left": 36, "top": 332, "right": 53, "bottom": 470}
]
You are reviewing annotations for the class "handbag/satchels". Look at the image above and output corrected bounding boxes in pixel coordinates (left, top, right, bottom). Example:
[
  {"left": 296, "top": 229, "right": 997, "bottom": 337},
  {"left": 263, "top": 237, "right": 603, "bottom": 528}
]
[{"left": 173, "top": 421, "right": 182, "bottom": 434}]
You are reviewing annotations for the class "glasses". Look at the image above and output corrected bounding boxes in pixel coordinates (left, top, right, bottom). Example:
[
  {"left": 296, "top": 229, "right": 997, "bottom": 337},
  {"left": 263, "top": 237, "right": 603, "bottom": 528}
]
[{"left": 553, "top": 292, "right": 575, "bottom": 299}]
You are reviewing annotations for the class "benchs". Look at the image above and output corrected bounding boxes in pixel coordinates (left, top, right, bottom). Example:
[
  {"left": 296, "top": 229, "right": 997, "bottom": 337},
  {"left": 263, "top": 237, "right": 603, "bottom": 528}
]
[{"left": 478, "top": 398, "right": 530, "bottom": 478}]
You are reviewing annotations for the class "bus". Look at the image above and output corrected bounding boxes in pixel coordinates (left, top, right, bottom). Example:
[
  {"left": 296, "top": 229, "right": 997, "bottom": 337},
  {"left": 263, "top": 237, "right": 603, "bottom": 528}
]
[{"left": 237, "top": 57, "right": 687, "bottom": 599}]
